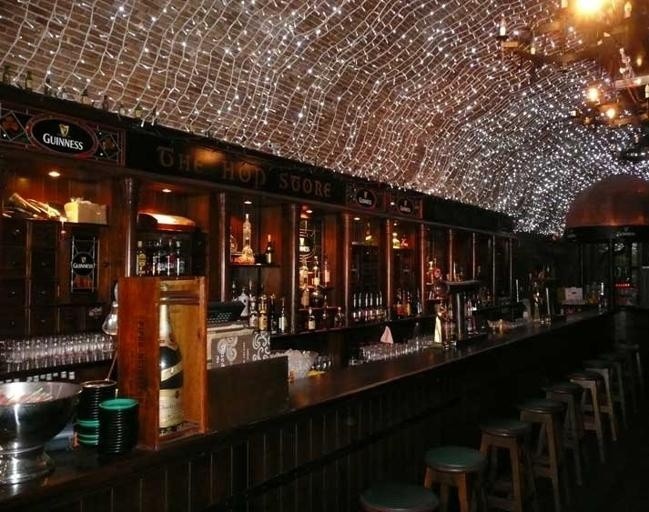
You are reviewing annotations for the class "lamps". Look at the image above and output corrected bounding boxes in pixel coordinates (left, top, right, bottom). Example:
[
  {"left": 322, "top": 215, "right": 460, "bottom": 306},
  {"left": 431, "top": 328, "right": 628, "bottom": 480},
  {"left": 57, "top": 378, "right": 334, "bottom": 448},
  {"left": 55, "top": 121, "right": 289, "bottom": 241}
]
[
  {"left": 495, "top": 1, "right": 648, "bottom": 83},
  {"left": 586, "top": 56, "right": 648, "bottom": 136}
]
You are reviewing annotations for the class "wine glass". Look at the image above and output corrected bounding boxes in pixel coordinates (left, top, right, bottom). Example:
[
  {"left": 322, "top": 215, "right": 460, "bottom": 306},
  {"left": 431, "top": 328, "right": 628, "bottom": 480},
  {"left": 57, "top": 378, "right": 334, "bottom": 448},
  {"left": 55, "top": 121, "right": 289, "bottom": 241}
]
[{"left": 1, "top": 380, "right": 82, "bottom": 484}]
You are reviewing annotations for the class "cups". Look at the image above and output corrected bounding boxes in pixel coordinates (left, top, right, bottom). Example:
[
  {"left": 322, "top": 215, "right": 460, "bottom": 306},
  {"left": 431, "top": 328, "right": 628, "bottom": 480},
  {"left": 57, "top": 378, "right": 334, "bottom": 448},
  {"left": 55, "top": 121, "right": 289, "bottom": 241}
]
[
  {"left": 309, "top": 338, "right": 433, "bottom": 372},
  {"left": 0, "top": 333, "right": 114, "bottom": 373}
]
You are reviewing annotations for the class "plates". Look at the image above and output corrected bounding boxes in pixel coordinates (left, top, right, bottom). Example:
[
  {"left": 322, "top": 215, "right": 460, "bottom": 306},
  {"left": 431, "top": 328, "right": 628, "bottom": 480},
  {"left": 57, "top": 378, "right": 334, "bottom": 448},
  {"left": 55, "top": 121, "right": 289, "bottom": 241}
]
[{"left": 79, "top": 378, "right": 140, "bottom": 454}]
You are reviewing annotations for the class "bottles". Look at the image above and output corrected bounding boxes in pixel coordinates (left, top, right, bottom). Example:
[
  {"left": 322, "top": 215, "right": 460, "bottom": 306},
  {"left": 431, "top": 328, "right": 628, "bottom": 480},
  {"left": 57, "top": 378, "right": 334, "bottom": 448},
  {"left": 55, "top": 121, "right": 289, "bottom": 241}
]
[
  {"left": 397, "top": 289, "right": 422, "bottom": 317},
  {"left": 296, "top": 255, "right": 345, "bottom": 331},
  {"left": 393, "top": 232, "right": 399, "bottom": 249},
  {"left": 158, "top": 284, "right": 183, "bottom": 434},
  {"left": 132, "top": 237, "right": 188, "bottom": 276},
  {"left": 232, "top": 281, "right": 288, "bottom": 336},
  {"left": 228, "top": 212, "right": 277, "bottom": 265},
  {"left": 400, "top": 232, "right": 410, "bottom": 247},
  {"left": 429, "top": 259, "right": 481, "bottom": 298},
  {"left": 352, "top": 290, "right": 387, "bottom": 323}
]
